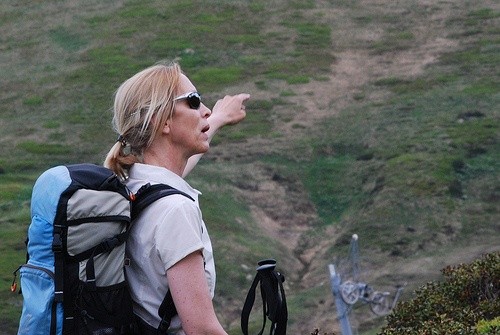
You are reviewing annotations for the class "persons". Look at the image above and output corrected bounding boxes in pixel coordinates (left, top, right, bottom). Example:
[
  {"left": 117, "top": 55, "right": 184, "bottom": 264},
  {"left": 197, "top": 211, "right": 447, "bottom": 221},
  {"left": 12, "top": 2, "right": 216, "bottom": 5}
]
[{"left": 100, "top": 59, "right": 250, "bottom": 335}]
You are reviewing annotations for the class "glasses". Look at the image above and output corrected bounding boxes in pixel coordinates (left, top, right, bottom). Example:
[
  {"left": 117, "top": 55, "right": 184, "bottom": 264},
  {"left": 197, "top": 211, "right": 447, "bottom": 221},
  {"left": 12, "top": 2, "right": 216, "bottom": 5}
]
[{"left": 169, "top": 91, "right": 200, "bottom": 109}]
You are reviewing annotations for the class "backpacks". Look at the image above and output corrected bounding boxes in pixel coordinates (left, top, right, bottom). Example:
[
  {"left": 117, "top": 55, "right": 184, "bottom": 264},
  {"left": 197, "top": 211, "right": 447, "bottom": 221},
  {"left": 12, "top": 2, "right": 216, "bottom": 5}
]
[{"left": 10, "top": 163, "right": 202, "bottom": 334}]
